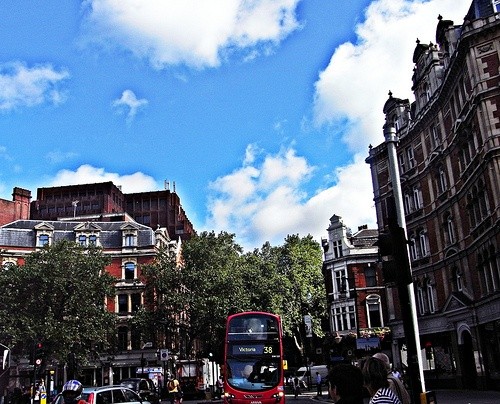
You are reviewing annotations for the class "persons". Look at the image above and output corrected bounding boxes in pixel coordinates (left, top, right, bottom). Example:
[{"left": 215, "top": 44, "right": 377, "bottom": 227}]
[
  {"left": 217, "top": 376, "right": 224, "bottom": 399},
  {"left": 315, "top": 372, "right": 322, "bottom": 395},
  {"left": 294, "top": 376, "right": 301, "bottom": 398},
  {"left": 62, "top": 380, "right": 90, "bottom": 404},
  {"left": 2, "top": 385, "right": 9, "bottom": 404},
  {"left": 167, "top": 374, "right": 183, "bottom": 404},
  {"left": 326, "top": 364, "right": 365, "bottom": 404},
  {"left": 248, "top": 367, "right": 265, "bottom": 383},
  {"left": 372, "top": 353, "right": 411, "bottom": 404},
  {"left": 392, "top": 368, "right": 401, "bottom": 381},
  {"left": 361, "top": 358, "right": 403, "bottom": 404}
]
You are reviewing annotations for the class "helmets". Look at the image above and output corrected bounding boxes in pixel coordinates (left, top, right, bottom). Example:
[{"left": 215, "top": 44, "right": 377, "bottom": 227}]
[{"left": 62, "top": 380, "right": 83, "bottom": 400}]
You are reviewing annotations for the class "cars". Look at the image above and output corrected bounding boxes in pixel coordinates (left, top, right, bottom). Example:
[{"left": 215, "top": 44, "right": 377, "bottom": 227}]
[{"left": 120, "top": 378, "right": 163, "bottom": 404}]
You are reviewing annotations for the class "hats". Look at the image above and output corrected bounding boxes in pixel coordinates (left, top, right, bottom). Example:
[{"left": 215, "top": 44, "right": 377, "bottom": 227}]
[
  {"left": 315, "top": 372, "right": 320, "bottom": 374},
  {"left": 171, "top": 374, "right": 175, "bottom": 378},
  {"left": 371, "top": 353, "right": 389, "bottom": 364}
]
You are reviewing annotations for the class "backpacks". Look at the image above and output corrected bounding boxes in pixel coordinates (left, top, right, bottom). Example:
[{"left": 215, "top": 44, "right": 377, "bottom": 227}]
[{"left": 167, "top": 380, "right": 174, "bottom": 390}]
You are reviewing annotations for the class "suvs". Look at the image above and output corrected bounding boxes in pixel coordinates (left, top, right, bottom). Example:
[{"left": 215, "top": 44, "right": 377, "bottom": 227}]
[{"left": 52, "top": 385, "right": 150, "bottom": 404}]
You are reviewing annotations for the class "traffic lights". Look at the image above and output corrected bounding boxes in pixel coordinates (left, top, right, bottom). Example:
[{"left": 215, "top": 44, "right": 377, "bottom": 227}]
[{"left": 370, "top": 226, "right": 413, "bottom": 287}]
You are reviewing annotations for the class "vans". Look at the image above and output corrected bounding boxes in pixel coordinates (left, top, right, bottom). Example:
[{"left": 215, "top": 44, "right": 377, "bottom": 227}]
[{"left": 294, "top": 365, "right": 330, "bottom": 387}]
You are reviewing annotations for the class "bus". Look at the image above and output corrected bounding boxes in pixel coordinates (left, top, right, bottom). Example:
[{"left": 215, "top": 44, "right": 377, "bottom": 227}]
[{"left": 223, "top": 310, "right": 288, "bottom": 404}]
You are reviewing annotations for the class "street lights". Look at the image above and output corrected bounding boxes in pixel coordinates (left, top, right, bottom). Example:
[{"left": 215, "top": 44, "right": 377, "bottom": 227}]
[{"left": 140, "top": 341, "right": 152, "bottom": 379}]
